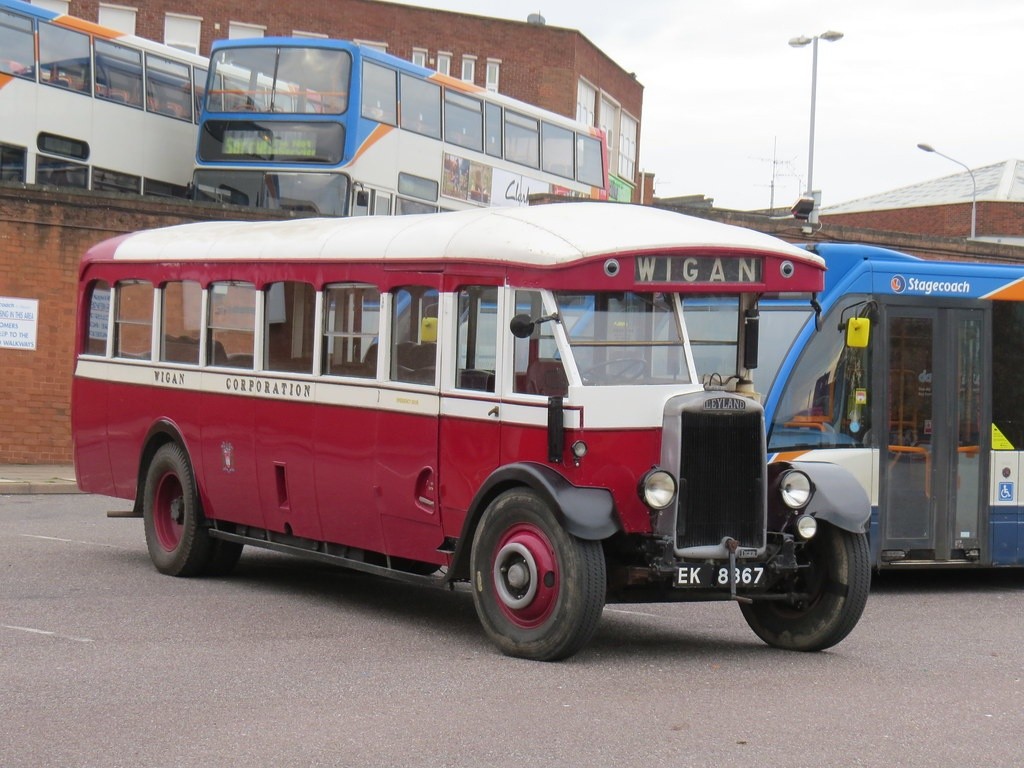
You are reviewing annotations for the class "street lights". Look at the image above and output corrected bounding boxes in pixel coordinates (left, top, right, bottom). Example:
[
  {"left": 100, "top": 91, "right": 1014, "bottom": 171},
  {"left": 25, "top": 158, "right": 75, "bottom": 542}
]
[
  {"left": 914, "top": 142, "right": 976, "bottom": 241},
  {"left": 788, "top": 31, "right": 846, "bottom": 224}
]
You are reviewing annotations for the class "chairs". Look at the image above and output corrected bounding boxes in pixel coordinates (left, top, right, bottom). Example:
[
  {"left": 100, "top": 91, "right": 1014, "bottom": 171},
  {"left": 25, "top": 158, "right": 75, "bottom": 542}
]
[
  {"left": 47, "top": 75, "right": 185, "bottom": 122},
  {"left": 327, "top": 339, "right": 495, "bottom": 393},
  {"left": 227, "top": 353, "right": 253, "bottom": 370},
  {"left": 525, "top": 357, "right": 570, "bottom": 397},
  {"left": 163, "top": 332, "right": 227, "bottom": 368}
]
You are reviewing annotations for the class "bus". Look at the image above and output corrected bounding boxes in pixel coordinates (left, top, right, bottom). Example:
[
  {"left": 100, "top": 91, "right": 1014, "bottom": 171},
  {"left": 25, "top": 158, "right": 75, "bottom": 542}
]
[
  {"left": 182, "top": 36, "right": 611, "bottom": 217},
  {"left": 325, "top": 239, "right": 1024, "bottom": 574},
  {"left": 1, "top": 0, "right": 384, "bottom": 195},
  {"left": 70, "top": 201, "right": 876, "bottom": 663}
]
